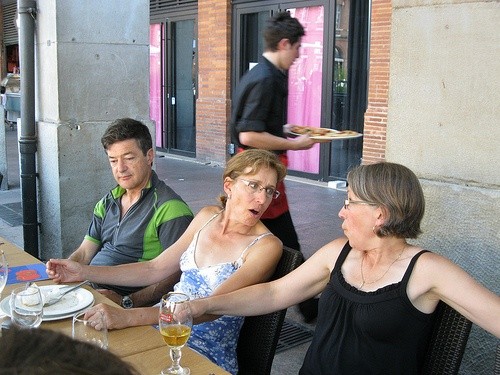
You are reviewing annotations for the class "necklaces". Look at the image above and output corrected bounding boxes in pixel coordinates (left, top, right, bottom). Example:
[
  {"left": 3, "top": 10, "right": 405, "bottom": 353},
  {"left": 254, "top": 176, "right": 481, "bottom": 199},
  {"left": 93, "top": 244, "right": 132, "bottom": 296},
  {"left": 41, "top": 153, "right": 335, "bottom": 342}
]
[{"left": 359, "top": 243, "right": 407, "bottom": 289}]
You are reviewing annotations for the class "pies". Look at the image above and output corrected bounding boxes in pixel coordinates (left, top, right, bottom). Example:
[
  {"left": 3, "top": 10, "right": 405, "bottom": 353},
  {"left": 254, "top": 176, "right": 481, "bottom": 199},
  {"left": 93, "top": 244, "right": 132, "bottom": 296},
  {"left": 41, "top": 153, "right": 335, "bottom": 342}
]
[{"left": 291, "top": 126, "right": 359, "bottom": 138}]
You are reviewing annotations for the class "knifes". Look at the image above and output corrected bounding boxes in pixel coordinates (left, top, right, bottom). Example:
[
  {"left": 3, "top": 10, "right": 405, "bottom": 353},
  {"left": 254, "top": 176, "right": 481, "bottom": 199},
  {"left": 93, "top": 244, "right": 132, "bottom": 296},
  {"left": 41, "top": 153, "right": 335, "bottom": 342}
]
[{"left": 43, "top": 279, "right": 89, "bottom": 307}]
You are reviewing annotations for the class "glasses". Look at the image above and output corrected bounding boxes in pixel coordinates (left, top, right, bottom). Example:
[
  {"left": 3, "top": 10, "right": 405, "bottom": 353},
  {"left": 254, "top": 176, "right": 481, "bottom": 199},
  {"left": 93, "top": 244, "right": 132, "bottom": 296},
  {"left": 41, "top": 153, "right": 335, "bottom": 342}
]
[
  {"left": 343, "top": 200, "right": 383, "bottom": 210},
  {"left": 237, "top": 177, "right": 280, "bottom": 199}
]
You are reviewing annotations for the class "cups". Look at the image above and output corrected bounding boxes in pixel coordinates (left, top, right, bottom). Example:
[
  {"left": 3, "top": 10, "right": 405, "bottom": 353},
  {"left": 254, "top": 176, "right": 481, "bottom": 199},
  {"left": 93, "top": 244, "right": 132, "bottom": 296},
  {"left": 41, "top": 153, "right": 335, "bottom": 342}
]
[
  {"left": 10, "top": 286, "right": 44, "bottom": 329},
  {"left": 72, "top": 310, "right": 109, "bottom": 350},
  {"left": 0, "top": 249, "right": 9, "bottom": 294}
]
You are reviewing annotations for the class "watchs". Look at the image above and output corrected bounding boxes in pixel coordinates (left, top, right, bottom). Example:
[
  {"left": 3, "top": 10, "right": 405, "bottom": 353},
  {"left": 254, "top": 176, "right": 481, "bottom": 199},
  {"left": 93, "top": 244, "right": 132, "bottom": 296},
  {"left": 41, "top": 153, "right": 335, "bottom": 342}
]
[{"left": 122, "top": 296, "right": 133, "bottom": 309}]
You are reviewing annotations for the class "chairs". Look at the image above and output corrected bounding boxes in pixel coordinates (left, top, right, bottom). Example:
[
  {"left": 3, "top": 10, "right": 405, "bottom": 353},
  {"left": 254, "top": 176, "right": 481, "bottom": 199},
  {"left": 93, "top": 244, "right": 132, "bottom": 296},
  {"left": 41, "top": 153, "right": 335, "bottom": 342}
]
[
  {"left": 432, "top": 300, "right": 474, "bottom": 375},
  {"left": 237, "top": 245, "right": 301, "bottom": 375}
]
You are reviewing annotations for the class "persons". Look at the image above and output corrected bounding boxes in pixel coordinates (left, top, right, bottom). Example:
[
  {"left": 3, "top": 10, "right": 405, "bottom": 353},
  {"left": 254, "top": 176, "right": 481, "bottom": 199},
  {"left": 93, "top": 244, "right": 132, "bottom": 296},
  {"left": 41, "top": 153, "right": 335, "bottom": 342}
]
[
  {"left": 169, "top": 162, "right": 500, "bottom": 375},
  {"left": 47, "top": 149, "right": 283, "bottom": 375},
  {"left": 230, "top": 12, "right": 320, "bottom": 324},
  {"left": 0, "top": 322, "right": 141, "bottom": 375},
  {"left": 66, "top": 118, "right": 195, "bottom": 309}
]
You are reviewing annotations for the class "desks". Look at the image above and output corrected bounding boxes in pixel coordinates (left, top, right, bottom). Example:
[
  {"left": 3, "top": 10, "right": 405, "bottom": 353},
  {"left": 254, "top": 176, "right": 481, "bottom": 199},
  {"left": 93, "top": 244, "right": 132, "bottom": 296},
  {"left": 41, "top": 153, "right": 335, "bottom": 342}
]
[{"left": 0, "top": 236, "right": 235, "bottom": 375}]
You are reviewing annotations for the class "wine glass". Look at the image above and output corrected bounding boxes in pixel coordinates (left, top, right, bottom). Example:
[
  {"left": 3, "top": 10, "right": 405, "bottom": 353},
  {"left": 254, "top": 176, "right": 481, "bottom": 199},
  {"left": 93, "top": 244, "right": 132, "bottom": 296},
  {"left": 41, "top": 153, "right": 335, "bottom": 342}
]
[{"left": 159, "top": 292, "right": 194, "bottom": 375}]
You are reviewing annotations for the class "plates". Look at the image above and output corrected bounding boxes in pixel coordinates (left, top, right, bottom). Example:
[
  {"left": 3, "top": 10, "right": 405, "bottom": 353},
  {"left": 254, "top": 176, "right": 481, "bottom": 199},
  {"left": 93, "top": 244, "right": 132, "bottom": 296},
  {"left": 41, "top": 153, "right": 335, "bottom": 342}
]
[
  {"left": 0, "top": 285, "right": 95, "bottom": 320},
  {"left": 284, "top": 127, "right": 363, "bottom": 141}
]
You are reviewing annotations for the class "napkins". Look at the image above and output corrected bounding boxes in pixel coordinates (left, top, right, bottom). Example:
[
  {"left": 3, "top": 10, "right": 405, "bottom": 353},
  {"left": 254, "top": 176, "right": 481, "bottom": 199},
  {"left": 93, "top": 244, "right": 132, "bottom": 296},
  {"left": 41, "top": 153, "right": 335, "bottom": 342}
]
[{"left": 0, "top": 263, "right": 52, "bottom": 284}]
